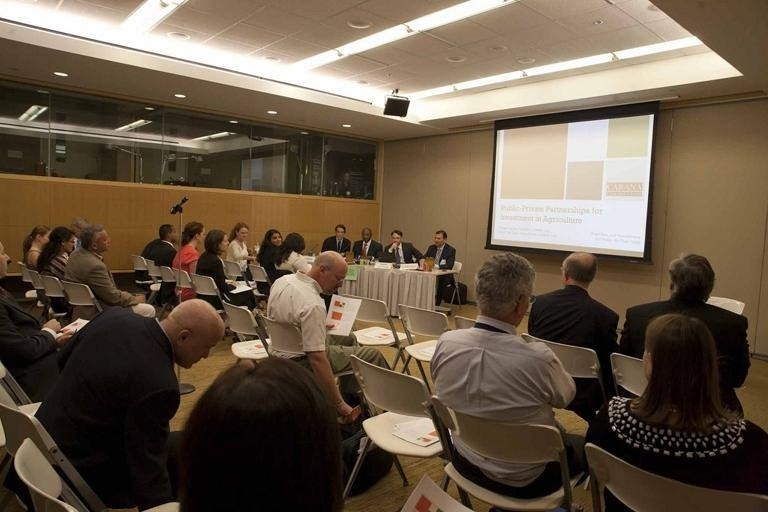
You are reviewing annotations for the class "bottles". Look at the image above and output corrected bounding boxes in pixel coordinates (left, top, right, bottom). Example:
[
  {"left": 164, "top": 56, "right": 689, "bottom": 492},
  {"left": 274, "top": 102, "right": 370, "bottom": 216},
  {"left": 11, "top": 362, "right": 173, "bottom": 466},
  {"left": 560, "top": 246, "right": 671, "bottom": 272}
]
[{"left": 424, "top": 257, "right": 435, "bottom": 272}]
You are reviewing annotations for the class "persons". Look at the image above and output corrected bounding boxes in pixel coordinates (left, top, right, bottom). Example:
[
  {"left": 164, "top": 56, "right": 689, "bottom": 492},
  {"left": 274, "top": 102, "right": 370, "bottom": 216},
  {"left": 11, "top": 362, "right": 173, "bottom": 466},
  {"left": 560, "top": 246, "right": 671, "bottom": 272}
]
[
  {"left": 352, "top": 227, "right": 384, "bottom": 259},
  {"left": 0, "top": 245, "right": 73, "bottom": 403},
  {"left": 265, "top": 251, "right": 391, "bottom": 417},
  {"left": 430, "top": 253, "right": 587, "bottom": 501},
  {"left": 585, "top": 314, "right": 766, "bottom": 511},
  {"left": 179, "top": 356, "right": 346, "bottom": 512},
  {"left": 338, "top": 171, "right": 356, "bottom": 200},
  {"left": 22, "top": 222, "right": 156, "bottom": 318},
  {"left": 384, "top": 230, "right": 424, "bottom": 264},
  {"left": 135, "top": 222, "right": 257, "bottom": 312},
  {"left": 423, "top": 230, "right": 456, "bottom": 306},
  {"left": 321, "top": 224, "right": 351, "bottom": 258},
  {"left": 255, "top": 229, "right": 312, "bottom": 296},
  {"left": 619, "top": 253, "right": 751, "bottom": 417},
  {"left": 39, "top": 298, "right": 225, "bottom": 512},
  {"left": 528, "top": 253, "right": 619, "bottom": 417}
]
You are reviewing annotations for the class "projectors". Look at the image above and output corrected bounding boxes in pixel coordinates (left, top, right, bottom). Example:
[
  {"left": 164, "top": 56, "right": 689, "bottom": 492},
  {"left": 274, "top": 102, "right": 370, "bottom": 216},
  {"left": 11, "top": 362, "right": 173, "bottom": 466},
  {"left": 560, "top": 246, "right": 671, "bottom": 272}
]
[{"left": 383, "top": 99, "right": 410, "bottom": 116}]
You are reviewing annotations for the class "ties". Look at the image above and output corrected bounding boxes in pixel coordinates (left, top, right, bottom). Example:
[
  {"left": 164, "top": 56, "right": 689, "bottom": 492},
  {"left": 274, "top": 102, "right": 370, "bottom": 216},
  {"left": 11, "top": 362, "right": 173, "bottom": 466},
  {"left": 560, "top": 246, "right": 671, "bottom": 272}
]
[
  {"left": 396, "top": 247, "right": 400, "bottom": 263},
  {"left": 338, "top": 240, "right": 341, "bottom": 253},
  {"left": 363, "top": 243, "right": 367, "bottom": 258}
]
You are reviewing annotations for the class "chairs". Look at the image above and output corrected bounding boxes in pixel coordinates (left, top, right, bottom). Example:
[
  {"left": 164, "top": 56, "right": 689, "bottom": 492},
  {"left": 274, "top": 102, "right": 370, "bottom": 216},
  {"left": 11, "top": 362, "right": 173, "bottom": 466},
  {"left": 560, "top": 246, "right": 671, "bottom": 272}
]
[
  {"left": 191, "top": 273, "right": 228, "bottom": 341},
  {"left": 222, "top": 300, "right": 274, "bottom": 369},
  {"left": 172, "top": 267, "right": 193, "bottom": 305},
  {"left": 248, "top": 264, "right": 273, "bottom": 308},
  {"left": 42, "top": 275, "right": 71, "bottom": 328},
  {"left": 340, "top": 353, "right": 472, "bottom": 508},
  {"left": 260, "top": 313, "right": 356, "bottom": 423},
  {"left": 396, "top": 302, "right": 452, "bottom": 395},
  {"left": 223, "top": 260, "right": 255, "bottom": 307},
  {"left": 146, "top": 260, "right": 163, "bottom": 304},
  {"left": 18, "top": 261, "right": 32, "bottom": 293},
  {"left": 133, "top": 256, "right": 163, "bottom": 306},
  {"left": 62, "top": 280, "right": 102, "bottom": 322},
  {"left": 520, "top": 331, "right": 605, "bottom": 416},
  {"left": 455, "top": 316, "right": 478, "bottom": 335},
  {"left": 340, "top": 292, "right": 414, "bottom": 378},
  {"left": 584, "top": 442, "right": 766, "bottom": 510},
  {"left": 13, "top": 435, "right": 181, "bottom": 511},
  {"left": 28, "top": 268, "right": 48, "bottom": 322},
  {"left": 158, "top": 267, "right": 178, "bottom": 321},
  {"left": 426, "top": 393, "right": 588, "bottom": 510},
  {"left": 0, "top": 385, "right": 104, "bottom": 508},
  {"left": 447, "top": 261, "right": 463, "bottom": 310},
  {"left": 2, "top": 364, "right": 39, "bottom": 420},
  {"left": 610, "top": 353, "right": 654, "bottom": 403}
]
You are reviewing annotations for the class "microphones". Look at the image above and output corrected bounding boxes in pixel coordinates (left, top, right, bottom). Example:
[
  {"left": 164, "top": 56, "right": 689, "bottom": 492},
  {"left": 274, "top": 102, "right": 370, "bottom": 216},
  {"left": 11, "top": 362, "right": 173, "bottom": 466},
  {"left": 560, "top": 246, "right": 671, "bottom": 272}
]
[{"left": 170, "top": 197, "right": 189, "bottom": 215}]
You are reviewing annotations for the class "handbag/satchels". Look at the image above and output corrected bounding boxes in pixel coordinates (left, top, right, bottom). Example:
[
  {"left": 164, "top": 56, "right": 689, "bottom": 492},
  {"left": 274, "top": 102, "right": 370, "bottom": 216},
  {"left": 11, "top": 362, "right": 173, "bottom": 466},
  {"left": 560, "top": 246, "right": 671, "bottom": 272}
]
[{"left": 339, "top": 430, "right": 393, "bottom": 497}]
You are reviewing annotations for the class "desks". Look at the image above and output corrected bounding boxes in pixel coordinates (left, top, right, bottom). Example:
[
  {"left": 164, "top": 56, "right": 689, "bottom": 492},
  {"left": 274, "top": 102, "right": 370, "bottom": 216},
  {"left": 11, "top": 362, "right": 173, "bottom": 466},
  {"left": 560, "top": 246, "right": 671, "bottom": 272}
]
[{"left": 303, "top": 255, "right": 459, "bottom": 317}]
